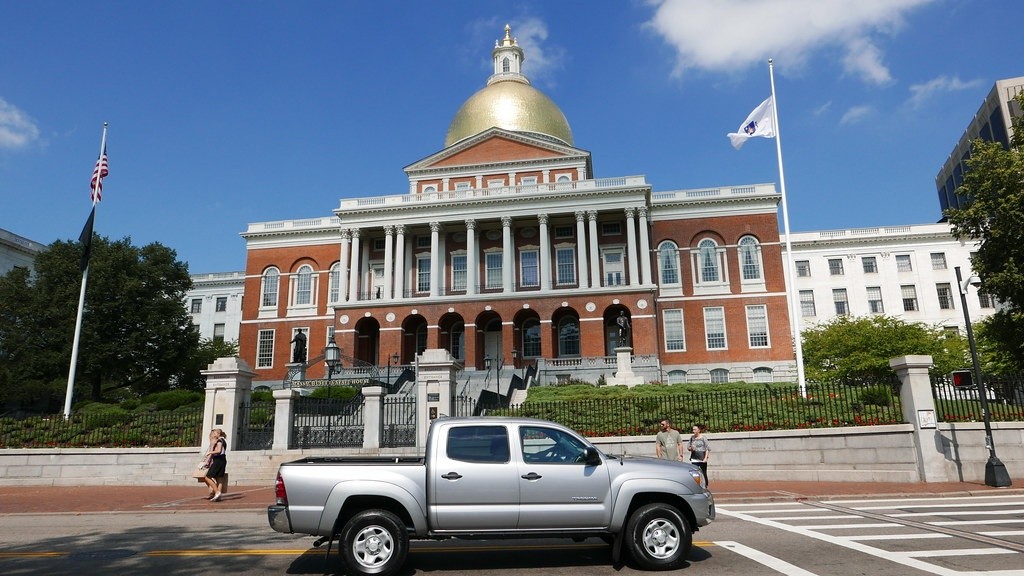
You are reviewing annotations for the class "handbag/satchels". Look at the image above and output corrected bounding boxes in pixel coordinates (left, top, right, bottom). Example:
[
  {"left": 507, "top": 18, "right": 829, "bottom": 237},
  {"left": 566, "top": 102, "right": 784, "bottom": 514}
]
[
  {"left": 193, "top": 462, "right": 210, "bottom": 478},
  {"left": 221, "top": 473, "right": 229, "bottom": 493}
]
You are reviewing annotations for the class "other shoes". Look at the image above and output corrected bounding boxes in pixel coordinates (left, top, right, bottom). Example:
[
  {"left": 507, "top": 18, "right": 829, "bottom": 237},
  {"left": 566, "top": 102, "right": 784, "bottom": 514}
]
[{"left": 210, "top": 492, "right": 222, "bottom": 502}]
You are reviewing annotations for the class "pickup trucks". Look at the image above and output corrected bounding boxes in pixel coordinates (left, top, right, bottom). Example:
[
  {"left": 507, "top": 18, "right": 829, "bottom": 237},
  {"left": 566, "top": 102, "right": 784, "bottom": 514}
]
[{"left": 268, "top": 416, "right": 716, "bottom": 576}]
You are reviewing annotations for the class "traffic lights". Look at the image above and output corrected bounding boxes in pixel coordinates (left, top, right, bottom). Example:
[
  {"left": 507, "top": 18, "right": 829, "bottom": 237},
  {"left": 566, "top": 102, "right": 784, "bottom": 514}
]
[{"left": 950, "top": 370, "right": 974, "bottom": 388}]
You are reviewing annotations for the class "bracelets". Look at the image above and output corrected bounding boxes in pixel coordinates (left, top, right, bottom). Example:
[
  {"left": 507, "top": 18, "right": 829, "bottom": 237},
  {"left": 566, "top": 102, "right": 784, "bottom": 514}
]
[{"left": 680, "top": 455, "right": 683, "bottom": 458}]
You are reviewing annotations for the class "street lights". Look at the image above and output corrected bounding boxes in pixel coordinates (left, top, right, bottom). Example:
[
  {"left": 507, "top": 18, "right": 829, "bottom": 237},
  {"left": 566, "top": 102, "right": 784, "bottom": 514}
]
[
  {"left": 324, "top": 335, "right": 341, "bottom": 447},
  {"left": 387, "top": 352, "right": 399, "bottom": 384},
  {"left": 511, "top": 347, "right": 518, "bottom": 374},
  {"left": 483, "top": 353, "right": 493, "bottom": 391}
]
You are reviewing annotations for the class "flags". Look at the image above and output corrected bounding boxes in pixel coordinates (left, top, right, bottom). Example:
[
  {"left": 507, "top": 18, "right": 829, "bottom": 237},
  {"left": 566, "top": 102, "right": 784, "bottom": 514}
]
[
  {"left": 79, "top": 206, "right": 94, "bottom": 273},
  {"left": 727, "top": 96, "right": 775, "bottom": 150},
  {"left": 91, "top": 142, "right": 109, "bottom": 203}
]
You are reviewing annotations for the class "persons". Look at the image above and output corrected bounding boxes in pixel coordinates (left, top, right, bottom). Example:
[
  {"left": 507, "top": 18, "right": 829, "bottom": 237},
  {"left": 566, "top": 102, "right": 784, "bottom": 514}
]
[
  {"left": 655, "top": 419, "right": 683, "bottom": 462},
  {"left": 616, "top": 311, "right": 631, "bottom": 347},
  {"left": 202, "top": 428, "right": 227, "bottom": 502},
  {"left": 687, "top": 425, "right": 711, "bottom": 487},
  {"left": 289, "top": 328, "right": 306, "bottom": 363}
]
